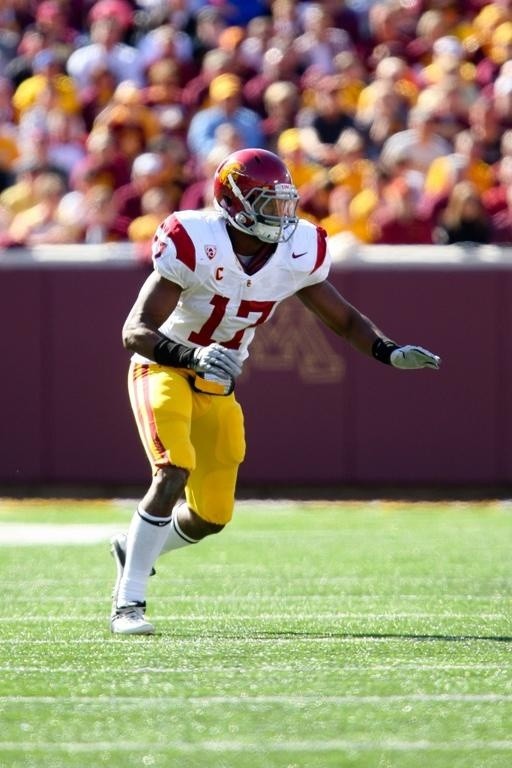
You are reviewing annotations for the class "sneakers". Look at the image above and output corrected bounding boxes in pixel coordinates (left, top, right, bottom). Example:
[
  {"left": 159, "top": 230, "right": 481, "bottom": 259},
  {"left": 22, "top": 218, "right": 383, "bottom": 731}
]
[
  {"left": 109, "top": 537, "right": 125, "bottom": 608},
  {"left": 110, "top": 605, "right": 153, "bottom": 634}
]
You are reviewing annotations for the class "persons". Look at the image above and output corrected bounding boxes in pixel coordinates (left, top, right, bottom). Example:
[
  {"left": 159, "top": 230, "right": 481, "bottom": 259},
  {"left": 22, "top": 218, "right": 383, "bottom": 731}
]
[
  {"left": 1, "top": 0, "right": 511, "bottom": 251},
  {"left": 108, "top": 146, "right": 441, "bottom": 637}
]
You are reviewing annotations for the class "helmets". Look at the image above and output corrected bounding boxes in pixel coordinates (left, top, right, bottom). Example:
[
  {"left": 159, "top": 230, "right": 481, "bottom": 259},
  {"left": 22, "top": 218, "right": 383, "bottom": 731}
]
[{"left": 215, "top": 148, "right": 297, "bottom": 243}]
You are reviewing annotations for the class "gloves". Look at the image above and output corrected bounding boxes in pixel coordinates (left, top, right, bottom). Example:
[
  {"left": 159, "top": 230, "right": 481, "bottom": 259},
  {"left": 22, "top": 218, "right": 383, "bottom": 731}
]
[
  {"left": 391, "top": 342, "right": 441, "bottom": 371},
  {"left": 193, "top": 347, "right": 243, "bottom": 379}
]
